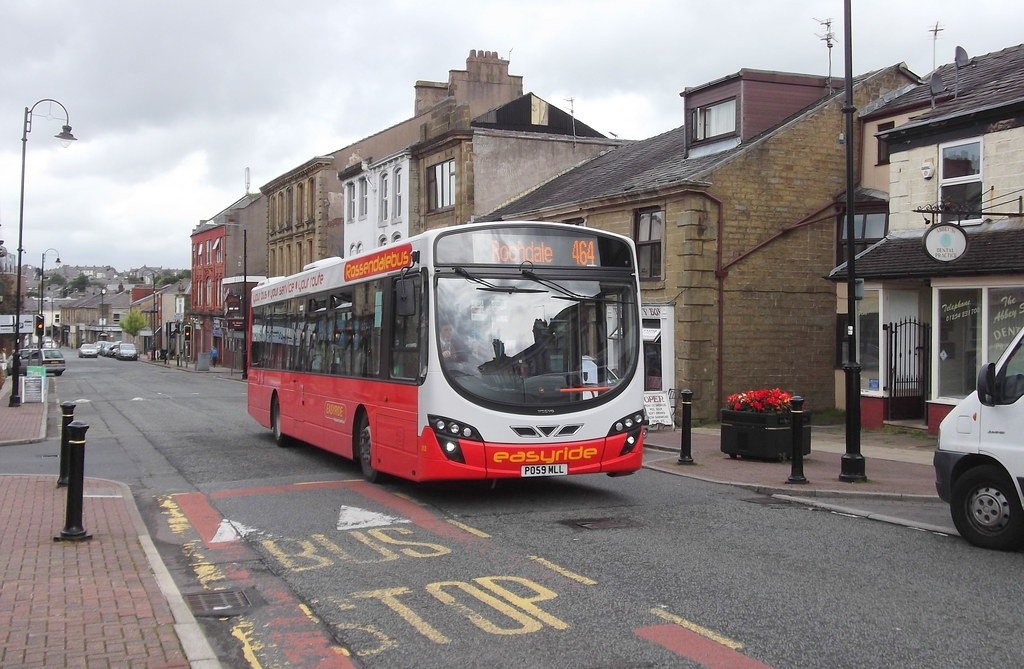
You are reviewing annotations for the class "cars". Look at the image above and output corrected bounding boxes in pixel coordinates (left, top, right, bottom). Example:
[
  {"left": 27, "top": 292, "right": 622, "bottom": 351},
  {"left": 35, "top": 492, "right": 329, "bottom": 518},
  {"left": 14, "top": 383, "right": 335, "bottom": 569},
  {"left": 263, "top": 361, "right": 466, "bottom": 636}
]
[
  {"left": 79, "top": 344, "right": 98, "bottom": 358},
  {"left": 94, "top": 341, "right": 122, "bottom": 357},
  {"left": 43, "top": 336, "right": 56, "bottom": 347},
  {"left": 116, "top": 343, "right": 137, "bottom": 361}
]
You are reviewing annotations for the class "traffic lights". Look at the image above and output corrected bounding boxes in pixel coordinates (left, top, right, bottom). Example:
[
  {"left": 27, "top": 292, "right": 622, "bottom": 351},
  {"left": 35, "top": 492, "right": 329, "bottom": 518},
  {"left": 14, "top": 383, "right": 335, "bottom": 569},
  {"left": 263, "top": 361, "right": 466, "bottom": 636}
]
[
  {"left": 35, "top": 317, "right": 44, "bottom": 336},
  {"left": 185, "top": 328, "right": 190, "bottom": 340}
]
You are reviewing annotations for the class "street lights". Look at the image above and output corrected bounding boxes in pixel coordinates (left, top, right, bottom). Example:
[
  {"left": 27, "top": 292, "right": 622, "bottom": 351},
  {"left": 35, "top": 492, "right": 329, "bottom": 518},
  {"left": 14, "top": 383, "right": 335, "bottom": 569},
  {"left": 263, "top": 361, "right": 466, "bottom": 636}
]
[
  {"left": 206, "top": 219, "right": 246, "bottom": 380},
  {"left": 40, "top": 248, "right": 61, "bottom": 313},
  {"left": 100, "top": 287, "right": 106, "bottom": 328},
  {"left": 9, "top": 100, "right": 78, "bottom": 409}
]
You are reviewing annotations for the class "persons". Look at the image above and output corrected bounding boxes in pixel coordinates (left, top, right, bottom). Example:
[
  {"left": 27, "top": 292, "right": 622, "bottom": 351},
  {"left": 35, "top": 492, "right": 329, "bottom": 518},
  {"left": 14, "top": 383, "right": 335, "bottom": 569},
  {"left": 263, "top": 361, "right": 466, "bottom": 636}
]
[
  {"left": 8, "top": 351, "right": 22, "bottom": 375},
  {"left": 210, "top": 344, "right": 218, "bottom": 368},
  {"left": 436, "top": 320, "right": 459, "bottom": 361},
  {"left": 0, "top": 349, "right": 10, "bottom": 377}
]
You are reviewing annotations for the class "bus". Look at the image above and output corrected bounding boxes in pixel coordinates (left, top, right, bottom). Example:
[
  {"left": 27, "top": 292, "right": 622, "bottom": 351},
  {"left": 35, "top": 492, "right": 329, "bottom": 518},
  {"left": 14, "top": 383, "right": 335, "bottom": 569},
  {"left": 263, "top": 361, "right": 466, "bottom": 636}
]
[{"left": 247, "top": 221, "right": 645, "bottom": 482}]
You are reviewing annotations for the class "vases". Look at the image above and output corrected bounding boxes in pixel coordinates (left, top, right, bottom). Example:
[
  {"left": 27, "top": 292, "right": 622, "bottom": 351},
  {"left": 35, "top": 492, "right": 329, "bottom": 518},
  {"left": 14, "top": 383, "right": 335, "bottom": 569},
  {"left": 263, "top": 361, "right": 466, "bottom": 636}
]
[{"left": 721, "top": 410, "right": 811, "bottom": 463}]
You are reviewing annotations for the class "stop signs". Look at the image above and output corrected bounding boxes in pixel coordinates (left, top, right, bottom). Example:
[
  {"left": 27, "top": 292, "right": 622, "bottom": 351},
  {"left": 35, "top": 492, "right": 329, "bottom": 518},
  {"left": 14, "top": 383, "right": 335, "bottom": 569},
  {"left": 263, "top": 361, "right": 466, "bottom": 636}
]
[{"left": 299, "top": 575, "right": 592, "bottom": 656}]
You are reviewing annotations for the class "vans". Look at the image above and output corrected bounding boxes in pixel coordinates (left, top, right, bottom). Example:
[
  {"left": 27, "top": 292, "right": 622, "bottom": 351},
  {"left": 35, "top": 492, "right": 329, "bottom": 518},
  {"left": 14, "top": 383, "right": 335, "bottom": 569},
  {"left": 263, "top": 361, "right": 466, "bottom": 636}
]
[
  {"left": 933, "top": 327, "right": 1024, "bottom": 548},
  {"left": 8, "top": 348, "right": 66, "bottom": 375}
]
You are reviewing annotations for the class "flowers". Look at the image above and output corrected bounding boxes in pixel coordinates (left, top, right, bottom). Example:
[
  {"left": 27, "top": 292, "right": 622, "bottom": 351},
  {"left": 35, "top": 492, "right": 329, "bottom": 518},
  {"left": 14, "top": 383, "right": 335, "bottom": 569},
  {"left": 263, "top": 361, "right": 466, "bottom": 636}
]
[{"left": 727, "top": 388, "right": 793, "bottom": 418}]
